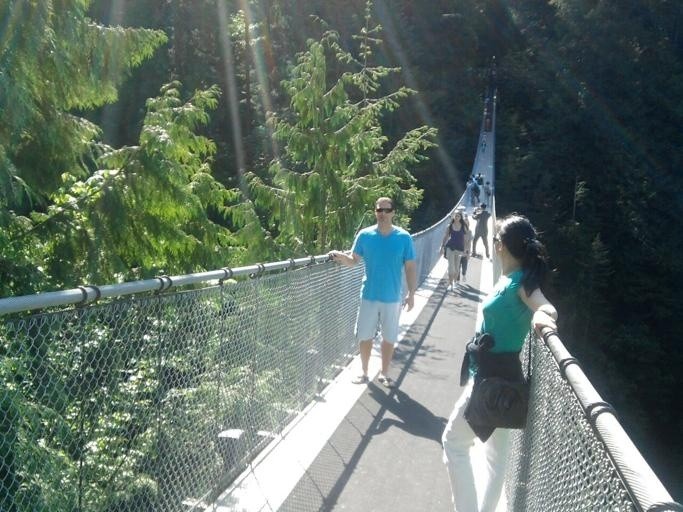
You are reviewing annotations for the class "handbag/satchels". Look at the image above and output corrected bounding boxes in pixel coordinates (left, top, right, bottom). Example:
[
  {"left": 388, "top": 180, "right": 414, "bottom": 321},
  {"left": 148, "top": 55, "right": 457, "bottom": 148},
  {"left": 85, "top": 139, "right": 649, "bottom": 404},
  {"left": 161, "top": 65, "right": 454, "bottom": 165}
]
[{"left": 464, "top": 375, "right": 530, "bottom": 429}]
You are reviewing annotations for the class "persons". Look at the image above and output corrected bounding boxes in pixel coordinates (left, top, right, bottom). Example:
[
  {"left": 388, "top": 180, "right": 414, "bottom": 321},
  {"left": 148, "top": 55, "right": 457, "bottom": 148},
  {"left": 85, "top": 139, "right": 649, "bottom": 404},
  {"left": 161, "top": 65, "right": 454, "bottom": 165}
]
[
  {"left": 330, "top": 197, "right": 417, "bottom": 388},
  {"left": 440, "top": 172, "right": 491, "bottom": 291},
  {"left": 480, "top": 55, "right": 496, "bottom": 154},
  {"left": 442, "top": 216, "right": 558, "bottom": 512}
]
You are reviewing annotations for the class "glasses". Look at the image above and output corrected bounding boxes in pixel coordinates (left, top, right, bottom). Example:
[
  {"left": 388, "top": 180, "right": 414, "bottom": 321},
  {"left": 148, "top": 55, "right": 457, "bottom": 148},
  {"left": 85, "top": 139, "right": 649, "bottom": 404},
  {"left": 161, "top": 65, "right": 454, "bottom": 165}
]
[{"left": 376, "top": 208, "right": 393, "bottom": 213}]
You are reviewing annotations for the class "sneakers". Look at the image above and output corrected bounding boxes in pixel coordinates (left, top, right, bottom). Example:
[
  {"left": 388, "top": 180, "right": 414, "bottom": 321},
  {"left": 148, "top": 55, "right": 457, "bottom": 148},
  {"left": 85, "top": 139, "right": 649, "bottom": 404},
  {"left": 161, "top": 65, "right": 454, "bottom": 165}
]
[
  {"left": 378, "top": 373, "right": 397, "bottom": 389},
  {"left": 351, "top": 374, "right": 369, "bottom": 384}
]
